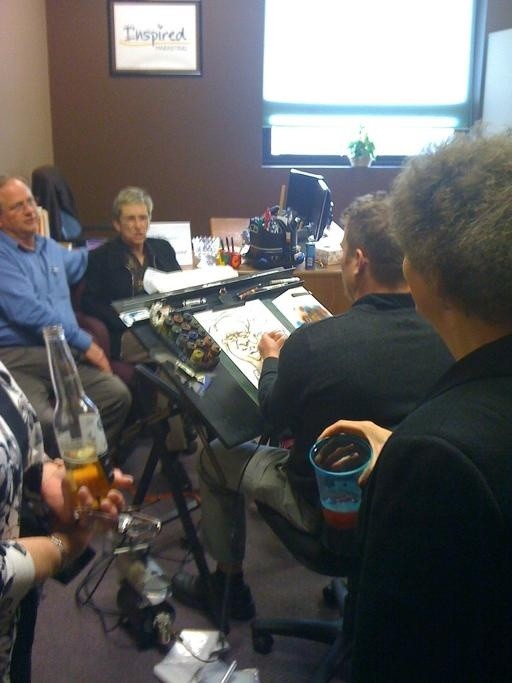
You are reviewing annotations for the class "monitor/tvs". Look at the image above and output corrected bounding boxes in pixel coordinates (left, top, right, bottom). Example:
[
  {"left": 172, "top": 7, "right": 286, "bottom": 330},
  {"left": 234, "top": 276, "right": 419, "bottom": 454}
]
[
  {"left": 286, "top": 169, "right": 322, "bottom": 221},
  {"left": 306, "top": 180, "right": 332, "bottom": 242}
]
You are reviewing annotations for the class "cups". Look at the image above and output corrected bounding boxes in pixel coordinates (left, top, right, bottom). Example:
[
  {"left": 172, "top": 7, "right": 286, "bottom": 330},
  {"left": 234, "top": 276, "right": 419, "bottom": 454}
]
[{"left": 309, "top": 433, "right": 375, "bottom": 531}]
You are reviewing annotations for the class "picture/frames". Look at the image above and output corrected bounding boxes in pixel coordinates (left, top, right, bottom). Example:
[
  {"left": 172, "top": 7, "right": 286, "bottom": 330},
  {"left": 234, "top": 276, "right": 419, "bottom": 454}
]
[{"left": 107, "top": 0, "right": 204, "bottom": 77}]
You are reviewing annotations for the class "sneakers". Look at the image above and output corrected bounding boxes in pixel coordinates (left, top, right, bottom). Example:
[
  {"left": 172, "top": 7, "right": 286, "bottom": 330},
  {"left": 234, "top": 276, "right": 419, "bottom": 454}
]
[{"left": 170, "top": 572, "right": 254, "bottom": 621}]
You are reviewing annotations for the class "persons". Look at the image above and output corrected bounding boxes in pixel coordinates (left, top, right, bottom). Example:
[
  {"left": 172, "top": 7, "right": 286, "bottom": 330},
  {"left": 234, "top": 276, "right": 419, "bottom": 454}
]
[
  {"left": 168, "top": 187, "right": 457, "bottom": 620},
  {"left": 311, "top": 129, "right": 510, "bottom": 683},
  {"left": 71, "top": 182, "right": 205, "bottom": 491},
  {"left": 0, "top": 356, "right": 136, "bottom": 682},
  {"left": 0, "top": 172, "right": 134, "bottom": 464}
]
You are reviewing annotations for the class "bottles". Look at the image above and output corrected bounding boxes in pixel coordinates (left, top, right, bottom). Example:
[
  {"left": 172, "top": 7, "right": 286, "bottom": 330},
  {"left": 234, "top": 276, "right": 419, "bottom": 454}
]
[
  {"left": 42, "top": 323, "right": 115, "bottom": 514},
  {"left": 306, "top": 235, "right": 317, "bottom": 270}
]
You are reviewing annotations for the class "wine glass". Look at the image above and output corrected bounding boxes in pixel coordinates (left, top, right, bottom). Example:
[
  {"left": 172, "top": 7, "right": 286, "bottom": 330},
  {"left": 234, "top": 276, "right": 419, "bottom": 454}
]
[{"left": 191, "top": 235, "right": 220, "bottom": 269}]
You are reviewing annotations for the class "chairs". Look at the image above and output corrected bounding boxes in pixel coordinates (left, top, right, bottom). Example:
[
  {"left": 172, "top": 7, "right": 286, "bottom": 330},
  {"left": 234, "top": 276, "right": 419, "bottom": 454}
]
[
  {"left": 251, "top": 471, "right": 378, "bottom": 683},
  {"left": 69, "top": 276, "right": 160, "bottom": 407}
]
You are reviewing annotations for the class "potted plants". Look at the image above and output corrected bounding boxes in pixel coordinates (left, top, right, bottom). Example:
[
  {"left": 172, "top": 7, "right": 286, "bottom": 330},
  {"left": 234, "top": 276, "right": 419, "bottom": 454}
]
[{"left": 346, "top": 123, "right": 377, "bottom": 168}]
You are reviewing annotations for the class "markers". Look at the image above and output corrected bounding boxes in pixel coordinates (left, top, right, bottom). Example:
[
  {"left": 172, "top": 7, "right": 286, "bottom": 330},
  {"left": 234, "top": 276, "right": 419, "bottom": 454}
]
[{"left": 182, "top": 297, "right": 207, "bottom": 307}]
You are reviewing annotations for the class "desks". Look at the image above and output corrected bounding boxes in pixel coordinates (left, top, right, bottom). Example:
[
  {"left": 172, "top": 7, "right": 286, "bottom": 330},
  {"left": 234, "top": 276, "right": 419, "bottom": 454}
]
[{"left": 210, "top": 217, "right": 353, "bottom": 316}]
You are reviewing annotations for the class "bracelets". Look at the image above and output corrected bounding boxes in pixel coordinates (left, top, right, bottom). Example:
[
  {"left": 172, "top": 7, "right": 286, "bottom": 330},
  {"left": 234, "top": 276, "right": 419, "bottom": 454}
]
[{"left": 47, "top": 533, "right": 67, "bottom": 576}]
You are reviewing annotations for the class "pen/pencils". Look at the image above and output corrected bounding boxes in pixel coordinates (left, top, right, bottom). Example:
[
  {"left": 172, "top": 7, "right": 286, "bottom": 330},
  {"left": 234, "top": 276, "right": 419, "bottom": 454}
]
[
  {"left": 234, "top": 276, "right": 300, "bottom": 301},
  {"left": 219, "top": 236, "right": 245, "bottom": 253}
]
